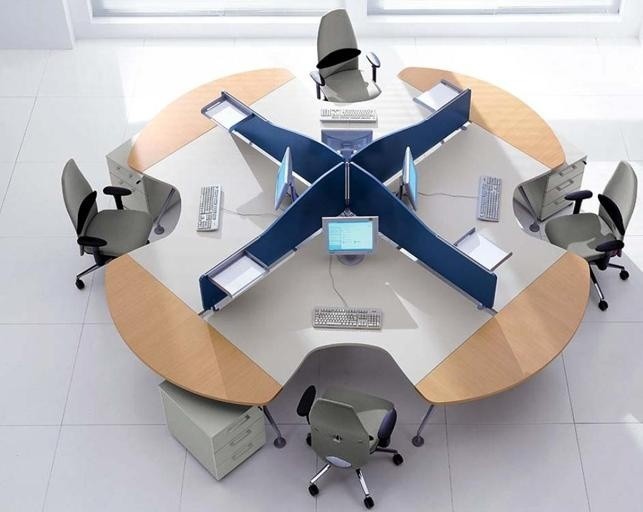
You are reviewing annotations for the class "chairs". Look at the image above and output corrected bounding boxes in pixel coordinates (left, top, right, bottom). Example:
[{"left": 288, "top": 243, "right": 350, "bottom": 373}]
[
  {"left": 295, "top": 384, "right": 403, "bottom": 510},
  {"left": 546, "top": 159, "right": 639, "bottom": 312},
  {"left": 310, "top": 9, "right": 381, "bottom": 103},
  {"left": 60, "top": 158, "right": 156, "bottom": 289}
]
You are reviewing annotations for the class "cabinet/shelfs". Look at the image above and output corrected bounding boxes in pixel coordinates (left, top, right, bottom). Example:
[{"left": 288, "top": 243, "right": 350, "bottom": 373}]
[
  {"left": 106, "top": 129, "right": 180, "bottom": 217},
  {"left": 514, "top": 132, "right": 587, "bottom": 221},
  {"left": 159, "top": 378, "right": 269, "bottom": 480}
]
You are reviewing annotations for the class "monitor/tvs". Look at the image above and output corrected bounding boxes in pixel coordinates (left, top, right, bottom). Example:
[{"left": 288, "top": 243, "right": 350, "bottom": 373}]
[
  {"left": 391, "top": 146, "right": 418, "bottom": 212},
  {"left": 321, "top": 128, "right": 373, "bottom": 157},
  {"left": 321, "top": 216, "right": 379, "bottom": 266},
  {"left": 274, "top": 147, "right": 297, "bottom": 211}
]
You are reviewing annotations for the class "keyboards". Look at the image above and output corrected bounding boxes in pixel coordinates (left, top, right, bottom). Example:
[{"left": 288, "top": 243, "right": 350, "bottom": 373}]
[
  {"left": 476, "top": 175, "right": 501, "bottom": 222},
  {"left": 196, "top": 185, "right": 221, "bottom": 231},
  {"left": 312, "top": 305, "right": 382, "bottom": 330},
  {"left": 319, "top": 108, "right": 377, "bottom": 123}
]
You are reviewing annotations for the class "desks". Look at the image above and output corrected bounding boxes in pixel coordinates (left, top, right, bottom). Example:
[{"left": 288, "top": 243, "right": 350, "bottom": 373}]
[{"left": 103, "top": 67, "right": 593, "bottom": 408}]
